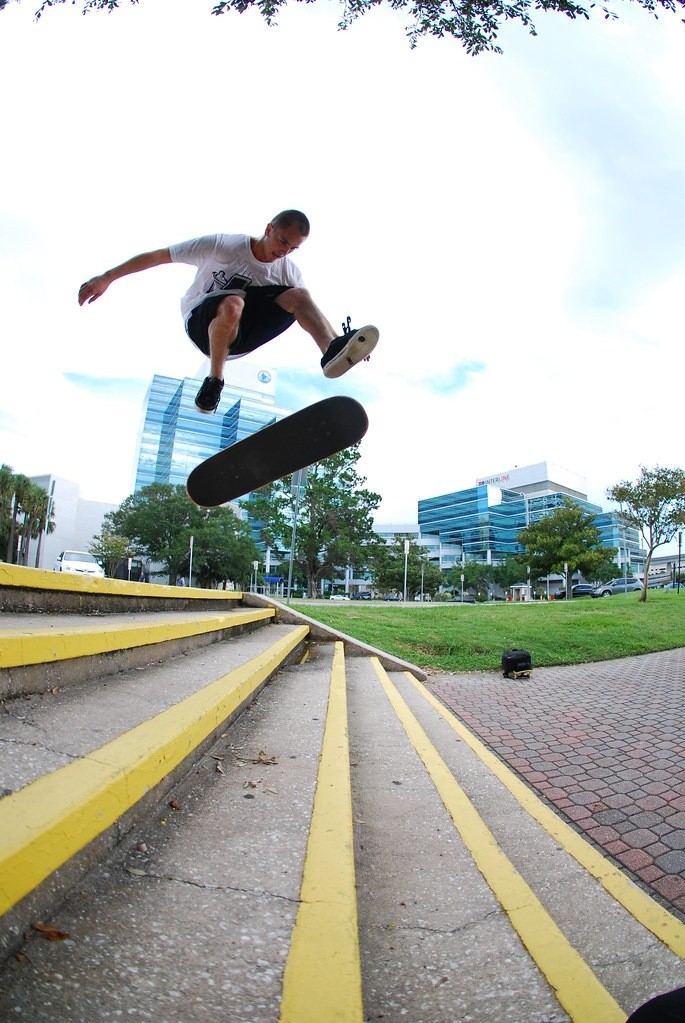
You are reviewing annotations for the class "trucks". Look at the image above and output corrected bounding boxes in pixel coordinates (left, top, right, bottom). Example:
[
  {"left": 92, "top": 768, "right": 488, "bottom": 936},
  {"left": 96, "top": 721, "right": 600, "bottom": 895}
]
[{"left": 372, "top": 589, "right": 403, "bottom": 599}]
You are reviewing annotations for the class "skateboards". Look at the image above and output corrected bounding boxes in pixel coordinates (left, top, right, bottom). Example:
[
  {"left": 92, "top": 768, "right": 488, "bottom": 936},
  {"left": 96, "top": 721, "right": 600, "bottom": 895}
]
[{"left": 187, "top": 396, "right": 368, "bottom": 507}]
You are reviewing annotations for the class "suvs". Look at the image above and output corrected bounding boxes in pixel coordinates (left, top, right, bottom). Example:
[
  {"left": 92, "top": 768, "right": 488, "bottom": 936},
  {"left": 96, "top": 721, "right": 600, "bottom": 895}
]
[
  {"left": 350, "top": 591, "right": 371, "bottom": 600},
  {"left": 414, "top": 594, "right": 431, "bottom": 602},
  {"left": 591, "top": 578, "right": 644, "bottom": 598},
  {"left": 554, "top": 584, "right": 593, "bottom": 600}
]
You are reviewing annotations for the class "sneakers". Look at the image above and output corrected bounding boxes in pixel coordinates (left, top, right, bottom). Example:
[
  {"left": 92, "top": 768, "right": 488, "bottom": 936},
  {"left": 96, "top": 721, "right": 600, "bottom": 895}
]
[
  {"left": 320, "top": 315, "right": 379, "bottom": 378},
  {"left": 195, "top": 375, "right": 225, "bottom": 415}
]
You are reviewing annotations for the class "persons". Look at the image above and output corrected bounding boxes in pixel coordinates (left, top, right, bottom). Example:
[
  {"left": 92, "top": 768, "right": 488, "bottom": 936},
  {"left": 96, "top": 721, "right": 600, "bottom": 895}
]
[
  {"left": 78, "top": 210, "right": 379, "bottom": 414},
  {"left": 139, "top": 572, "right": 145, "bottom": 582},
  {"left": 178, "top": 577, "right": 185, "bottom": 586}
]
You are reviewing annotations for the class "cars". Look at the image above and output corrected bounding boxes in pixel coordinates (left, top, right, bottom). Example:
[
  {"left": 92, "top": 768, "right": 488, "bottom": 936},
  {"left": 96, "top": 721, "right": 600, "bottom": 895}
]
[
  {"left": 384, "top": 594, "right": 401, "bottom": 602},
  {"left": 53, "top": 550, "right": 105, "bottom": 578},
  {"left": 330, "top": 595, "right": 350, "bottom": 600},
  {"left": 666, "top": 583, "right": 684, "bottom": 589},
  {"left": 452, "top": 595, "right": 475, "bottom": 603}
]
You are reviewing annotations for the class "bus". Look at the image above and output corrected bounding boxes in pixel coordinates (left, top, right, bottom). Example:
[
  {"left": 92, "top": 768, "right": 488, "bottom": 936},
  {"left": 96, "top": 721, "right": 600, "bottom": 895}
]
[{"left": 92, "top": 553, "right": 141, "bottom": 581}]
